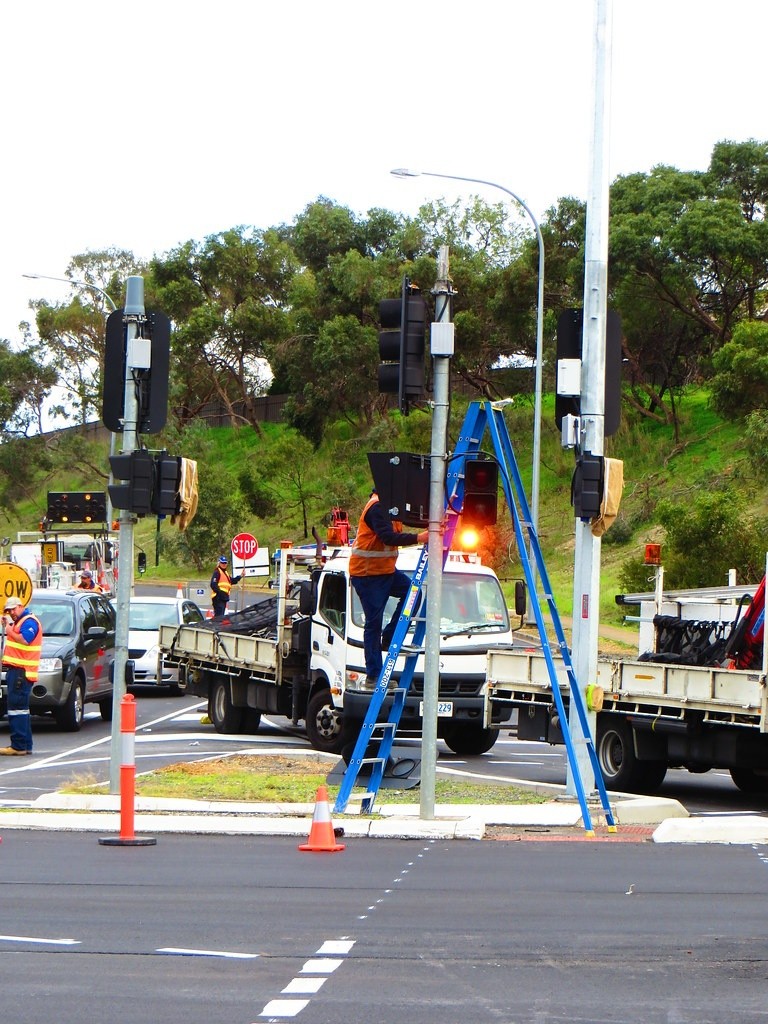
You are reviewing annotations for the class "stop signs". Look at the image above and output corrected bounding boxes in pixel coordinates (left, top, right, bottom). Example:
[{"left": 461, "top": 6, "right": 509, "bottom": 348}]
[{"left": 231, "top": 532, "right": 259, "bottom": 561}]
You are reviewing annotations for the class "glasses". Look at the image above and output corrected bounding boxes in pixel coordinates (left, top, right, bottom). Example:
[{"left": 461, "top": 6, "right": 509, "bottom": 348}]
[
  {"left": 221, "top": 563, "right": 227, "bottom": 564},
  {"left": 80, "top": 577, "right": 87, "bottom": 579}
]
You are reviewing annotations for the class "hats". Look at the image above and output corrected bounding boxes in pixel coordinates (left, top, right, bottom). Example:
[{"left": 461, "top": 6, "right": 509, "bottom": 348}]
[
  {"left": 218, "top": 556, "right": 228, "bottom": 563},
  {"left": 79, "top": 571, "right": 92, "bottom": 578},
  {"left": 3, "top": 596, "right": 23, "bottom": 612}
]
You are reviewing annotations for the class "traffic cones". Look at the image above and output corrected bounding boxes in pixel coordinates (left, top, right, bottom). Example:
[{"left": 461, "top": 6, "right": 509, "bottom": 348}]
[
  {"left": 224, "top": 603, "right": 229, "bottom": 616},
  {"left": 205, "top": 610, "right": 213, "bottom": 620},
  {"left": 297, "top": 786, "right": 346, "bottom": 853},
  {"left": 175, "top": 582, "right": 185, "bottom": 599}
]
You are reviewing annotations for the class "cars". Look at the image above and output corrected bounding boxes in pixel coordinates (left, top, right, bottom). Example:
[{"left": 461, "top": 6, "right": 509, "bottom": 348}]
[
  {"left": 97, "top": 595, "right": 205, "bottom": 697},
  {"left": 0, "top": 588, "right": 117, "bottom": 731}
]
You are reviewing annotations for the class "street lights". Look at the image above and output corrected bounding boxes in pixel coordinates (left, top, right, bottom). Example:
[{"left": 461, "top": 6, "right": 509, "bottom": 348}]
[
  {"left": 22, "top": 272, "right": 119, "bottom": 545},
  {"left": 388, "top": 165, "right": 546, "bottom": 627}
]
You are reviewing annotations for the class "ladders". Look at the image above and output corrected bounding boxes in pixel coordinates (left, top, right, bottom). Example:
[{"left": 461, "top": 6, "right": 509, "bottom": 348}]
[{"left": 335, "top": 401, "right": 619, "bottom": 838}]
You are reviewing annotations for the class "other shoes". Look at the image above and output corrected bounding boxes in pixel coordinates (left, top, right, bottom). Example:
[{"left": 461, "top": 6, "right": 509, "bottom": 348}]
[
  {"left": 383, "top": 642, "right": 392, "bottom": 651},
  {"left": 0, "top": 746, "right": 27, "bottom": 756},
  {"left": 27, "top": 750, "right": 33, "bottom": 754},
  {"left": 364, "top": 676, "right": 398, "bottom": 689}
]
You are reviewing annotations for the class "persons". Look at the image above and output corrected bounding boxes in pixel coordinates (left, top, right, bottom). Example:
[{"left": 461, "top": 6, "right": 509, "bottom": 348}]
[
  {"left": 349, "top": 486, "right": 430, "bottom": 690},
  {"left": 76, "top": 571, "right": 103, "bottom": 594},
  {"left": 0, "top": 598, "right": 43, "bottom": 756},
  {"left": 209, "top": 556, "right": 246, "bottom": 620}
]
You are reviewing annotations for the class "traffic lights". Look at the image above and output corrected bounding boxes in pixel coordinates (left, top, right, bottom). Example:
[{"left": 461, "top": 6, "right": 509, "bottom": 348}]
[
  {"left": 108, "top": 450, "right": 156, "bottom": 517},
  {"left": 377, "top": 270, "right": 426, "bottom": 416}
]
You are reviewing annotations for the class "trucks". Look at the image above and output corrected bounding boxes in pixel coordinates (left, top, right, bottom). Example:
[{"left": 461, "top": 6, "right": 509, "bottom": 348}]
[
  {"left": 155, "top": 502, "right": 527, "bottom": 758},
  {"left": 481, "top": 542, "right": 768, "bottom": 806},
  {"left": 0, "top": 490, "right": 147, "bottom": 600}
]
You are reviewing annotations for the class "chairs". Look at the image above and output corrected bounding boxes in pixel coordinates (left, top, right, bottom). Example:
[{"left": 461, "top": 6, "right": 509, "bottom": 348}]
[{"left": 39, "top": 611, "right": 71, "bottom": 634}]
